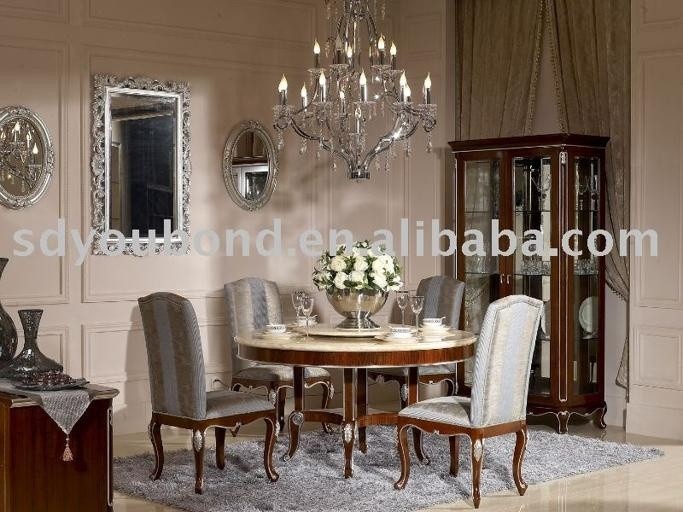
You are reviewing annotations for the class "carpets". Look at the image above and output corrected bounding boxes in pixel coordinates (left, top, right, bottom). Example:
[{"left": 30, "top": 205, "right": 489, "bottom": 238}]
[{"left": 113, "top": 420, "right": 666, "bottom": 512}]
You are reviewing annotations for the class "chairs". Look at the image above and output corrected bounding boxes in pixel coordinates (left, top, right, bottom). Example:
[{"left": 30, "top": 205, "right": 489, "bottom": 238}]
[
  {"left": 135, "top": 291, "right": 279, "bottom": 496},
  {"left": 219, "top": 275, "right": 333, "bottom": 438},
  {"left": 391, "top": 293, "right": 546, "bottom": 510},
  {"left": 366, "top": 275, "right": 466, "bottom": 411}
]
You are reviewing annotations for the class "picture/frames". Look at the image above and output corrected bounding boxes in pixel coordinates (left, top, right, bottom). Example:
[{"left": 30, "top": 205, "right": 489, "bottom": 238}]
[{"left": 325, "top": 285, "right": 389, "bottom": 330}]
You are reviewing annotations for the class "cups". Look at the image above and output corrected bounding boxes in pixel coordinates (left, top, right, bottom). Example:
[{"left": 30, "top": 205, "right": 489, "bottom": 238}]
[
  {"left": 392, "top": 327, "right": 409, "bottom": 335},
  {"left": 422, "top": 316, "right": 448, "bottom": 328},
  {"left": 513, "top": 251, "right": 598, "bottom": 273},
  {"left": 266, "top": 323, "right": 287, "bottom": 333}
]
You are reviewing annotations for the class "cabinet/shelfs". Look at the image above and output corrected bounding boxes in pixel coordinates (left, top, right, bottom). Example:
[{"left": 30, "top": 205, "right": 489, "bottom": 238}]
[
  {"left": 0, "top": 378, "right": 120, "bottom": 512},
  {"left": 445, "top": 132, "right": 612, "bottom": 437},
  {"left": 230, "top": 132, "right": 268, "bottom": 200}
]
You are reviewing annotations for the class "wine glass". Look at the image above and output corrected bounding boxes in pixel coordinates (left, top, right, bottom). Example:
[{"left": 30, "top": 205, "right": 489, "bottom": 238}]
[
  {"left": 291, "top": 290, "right": 316, "bottom": 342},
  {"left": 524, "top": 167, "right": 599, "bottom": 211},
  {"left": 395, "top": 290, "right": 425, "bottom": 337}
]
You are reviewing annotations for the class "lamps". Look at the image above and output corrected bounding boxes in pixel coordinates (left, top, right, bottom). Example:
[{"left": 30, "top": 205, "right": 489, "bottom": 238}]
[
  {"left": 269, "top": 0, "right": 442, "bottom": 185},
  {"left": 0, "top": 117, "right": 41, "bottom": 193}
]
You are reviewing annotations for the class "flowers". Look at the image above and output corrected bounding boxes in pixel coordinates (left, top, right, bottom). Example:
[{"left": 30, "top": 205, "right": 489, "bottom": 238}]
[{"left": 311, "top": 239, "right": 404, "bottom": 293}]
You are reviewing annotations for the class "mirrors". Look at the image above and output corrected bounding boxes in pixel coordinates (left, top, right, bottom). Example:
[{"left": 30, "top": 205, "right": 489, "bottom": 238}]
[
  {"left": 85, "top": 71, "right": 192, "bottom": 257},
  {"left": 0, "top": 103, "right": 55, "bottom": 212},
  {"left": 222, "top": 119, "right": 280, "bottom": 212}
]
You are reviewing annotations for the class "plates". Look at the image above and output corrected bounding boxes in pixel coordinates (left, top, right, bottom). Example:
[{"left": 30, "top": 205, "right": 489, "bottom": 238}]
[
  {"left": 417, "top": 326, "right": 449, "bottom": 336},
  {"left": 540, "top": 300, "right": 551, "bottom": 335},
  {"left": 13, "top": 380, "right": 88, "bottom": 390},
  {"left": 578, "top": 296, "right": 599, "bottom": 336},
  {"left": 258, "top": 332, "right": 292, "bottom": 340},
  {"left": 376, "top": 333, "right": 415, "bottom": 344}
]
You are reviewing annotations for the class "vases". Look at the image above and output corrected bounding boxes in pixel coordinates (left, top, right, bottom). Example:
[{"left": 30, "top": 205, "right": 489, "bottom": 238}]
[
  {"left": 0, "top": 257, "right": 17, "bottom": 366},
  {"left": 1, "top": 308, "right": 63, "bottom": 385}
]
